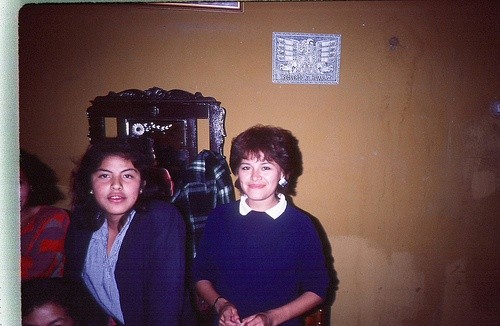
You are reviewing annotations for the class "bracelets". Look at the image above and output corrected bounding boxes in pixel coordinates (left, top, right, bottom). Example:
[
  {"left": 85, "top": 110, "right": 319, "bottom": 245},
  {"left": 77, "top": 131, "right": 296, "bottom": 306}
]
[{"left": 212, "top": 296, "right": 223, "bottom": 311}]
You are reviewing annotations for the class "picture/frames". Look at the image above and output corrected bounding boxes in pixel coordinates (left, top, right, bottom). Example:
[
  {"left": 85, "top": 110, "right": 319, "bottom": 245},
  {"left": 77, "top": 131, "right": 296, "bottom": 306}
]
[{"left": 88, "top": 3, "right": 245, "bottom": 14}]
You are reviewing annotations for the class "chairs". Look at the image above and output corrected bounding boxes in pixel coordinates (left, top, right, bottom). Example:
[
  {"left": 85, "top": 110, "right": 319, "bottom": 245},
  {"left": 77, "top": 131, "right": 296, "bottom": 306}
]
[{"left": 87, "top": 87, "right": 326, "bottom": 325}]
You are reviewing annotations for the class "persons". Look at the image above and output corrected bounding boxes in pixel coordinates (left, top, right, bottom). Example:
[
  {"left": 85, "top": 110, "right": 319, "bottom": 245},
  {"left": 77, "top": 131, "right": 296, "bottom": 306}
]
[
  {"left": 193, "top": 125, "right": 331, "bottom": 326},
  {"left": 20, "top": 148, "right": 73, "bottom": 284},
  {"left": 21, "top": 277, "right": 85, "bottom": 326},
  {"left": 63, "top": 137, "right": 186, "bottom": 326}
]
